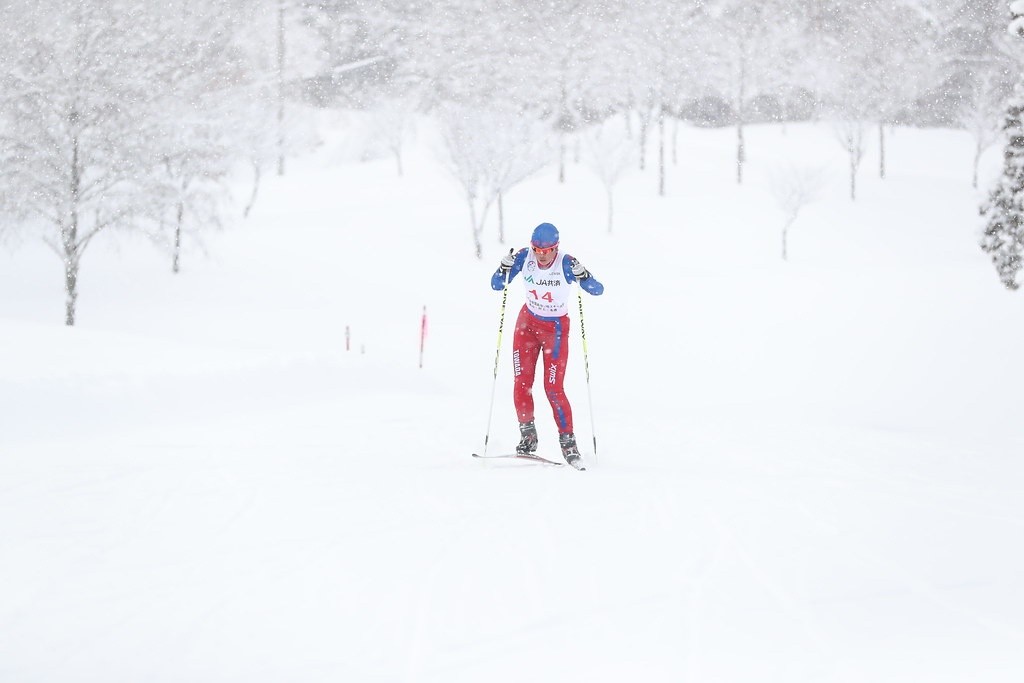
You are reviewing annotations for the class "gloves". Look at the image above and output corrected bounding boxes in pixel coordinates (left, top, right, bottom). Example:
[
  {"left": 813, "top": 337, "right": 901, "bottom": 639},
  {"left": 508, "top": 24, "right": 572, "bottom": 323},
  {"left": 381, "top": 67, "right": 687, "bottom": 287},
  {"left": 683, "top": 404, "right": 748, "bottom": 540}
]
[
  {"left": 569, "top": 257, "right": 588, "bottom": 279},
  {"left": 499, "top": 248, "right": 516, "bottom": 273}
]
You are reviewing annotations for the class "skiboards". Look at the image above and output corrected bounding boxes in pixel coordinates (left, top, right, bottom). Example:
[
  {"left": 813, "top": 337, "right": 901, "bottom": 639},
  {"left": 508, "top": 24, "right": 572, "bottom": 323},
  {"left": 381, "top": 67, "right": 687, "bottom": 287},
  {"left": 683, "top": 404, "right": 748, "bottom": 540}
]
[{"left": 471, "top": 452, "right": 587, "bottom": 472}]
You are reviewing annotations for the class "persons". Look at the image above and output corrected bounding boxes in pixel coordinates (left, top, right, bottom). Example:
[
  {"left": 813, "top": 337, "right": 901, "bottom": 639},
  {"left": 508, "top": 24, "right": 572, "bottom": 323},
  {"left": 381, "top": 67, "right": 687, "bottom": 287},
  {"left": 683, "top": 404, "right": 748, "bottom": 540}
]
[{"left": 490, "top": 222, "right": 605, "bottom": 464}]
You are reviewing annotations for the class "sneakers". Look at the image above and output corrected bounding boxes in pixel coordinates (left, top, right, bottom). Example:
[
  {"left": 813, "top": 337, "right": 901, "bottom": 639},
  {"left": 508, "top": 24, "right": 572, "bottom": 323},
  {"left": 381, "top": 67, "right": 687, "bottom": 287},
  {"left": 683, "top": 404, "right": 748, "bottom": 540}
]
[
  {"left": 559, "top": 432, "right": 585, "bottom": 471},
  {"left": 515, "top": 422, "right": 538, "bottom": 457}
]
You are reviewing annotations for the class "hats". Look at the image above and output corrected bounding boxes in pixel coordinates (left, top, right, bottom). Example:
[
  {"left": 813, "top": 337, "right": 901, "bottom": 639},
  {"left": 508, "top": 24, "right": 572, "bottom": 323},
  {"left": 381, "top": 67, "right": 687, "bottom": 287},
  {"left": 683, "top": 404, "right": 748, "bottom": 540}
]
[{"left": 531, "top": 223, "right": 559, "bottom": 249}]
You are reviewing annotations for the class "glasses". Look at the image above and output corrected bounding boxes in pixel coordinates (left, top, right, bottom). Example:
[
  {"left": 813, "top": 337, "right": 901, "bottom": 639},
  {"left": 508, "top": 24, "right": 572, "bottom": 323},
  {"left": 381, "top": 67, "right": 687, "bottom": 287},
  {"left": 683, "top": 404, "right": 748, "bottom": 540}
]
[{"left": 530, "top": 241, "right": 558, "bottom": 255}]
